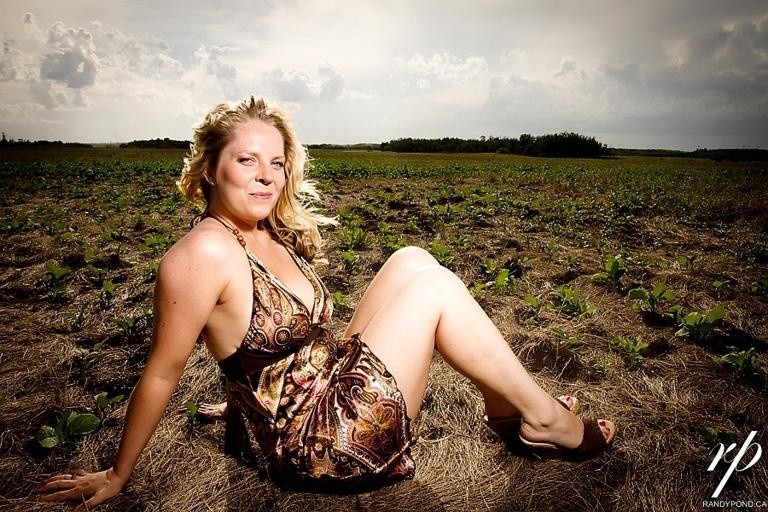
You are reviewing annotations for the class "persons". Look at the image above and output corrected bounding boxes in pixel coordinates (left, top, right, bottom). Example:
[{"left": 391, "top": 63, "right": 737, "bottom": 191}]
[{"left": 41, "top": 96, "right": 618, "bottom": 511}]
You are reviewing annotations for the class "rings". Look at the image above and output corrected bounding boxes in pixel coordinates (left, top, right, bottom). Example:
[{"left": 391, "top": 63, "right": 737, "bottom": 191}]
[{"left": 71, "top": 472, "right": 77, "bottom": 479}]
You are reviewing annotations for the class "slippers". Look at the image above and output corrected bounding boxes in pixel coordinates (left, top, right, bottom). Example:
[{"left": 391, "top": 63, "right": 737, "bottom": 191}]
[{"left": 483, "top": 395, "right": 616, "bottom": 450}]
